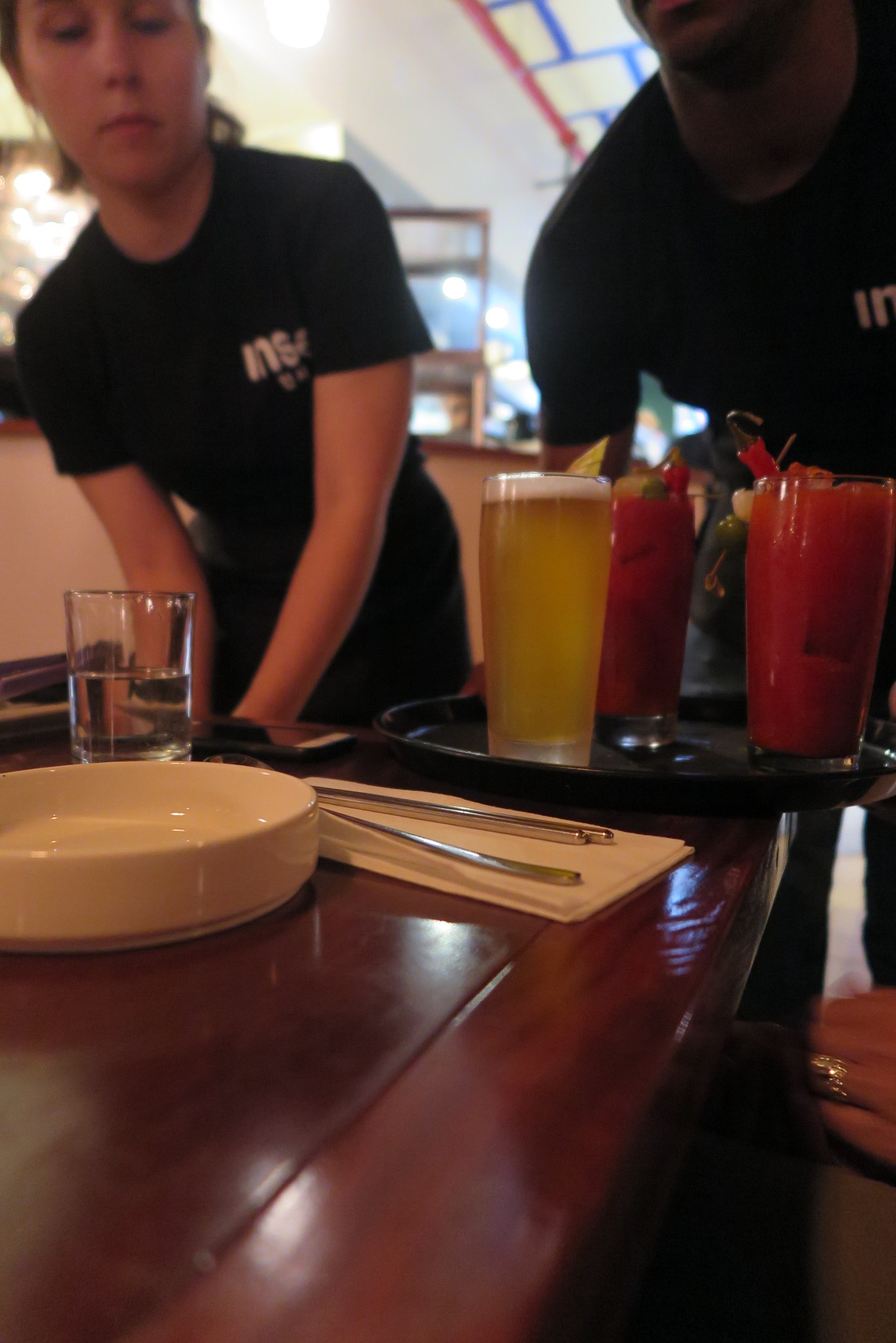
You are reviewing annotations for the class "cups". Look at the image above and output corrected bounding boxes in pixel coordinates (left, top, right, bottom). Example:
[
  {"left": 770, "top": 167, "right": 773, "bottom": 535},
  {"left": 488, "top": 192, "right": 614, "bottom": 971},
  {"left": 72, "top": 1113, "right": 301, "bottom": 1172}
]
[
  {"left": 476, "top": 456, "right": 896, "bottom": 775},
  {"left": 59, "top": 588, "right": 196, "bottom": 764}
]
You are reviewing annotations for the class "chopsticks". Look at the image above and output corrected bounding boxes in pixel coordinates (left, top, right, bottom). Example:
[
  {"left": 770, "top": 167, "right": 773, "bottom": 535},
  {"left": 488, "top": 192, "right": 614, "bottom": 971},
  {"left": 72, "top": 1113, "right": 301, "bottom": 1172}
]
[{"left": 311, "top": 783, "right": 615, "bottom": 845}]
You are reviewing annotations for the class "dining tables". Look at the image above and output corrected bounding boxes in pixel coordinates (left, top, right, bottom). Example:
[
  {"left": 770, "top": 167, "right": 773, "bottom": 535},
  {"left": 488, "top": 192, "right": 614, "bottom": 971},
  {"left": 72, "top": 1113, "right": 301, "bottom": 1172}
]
[{"left": 0, "top": 719, "right": 809, "bottom": 1341}]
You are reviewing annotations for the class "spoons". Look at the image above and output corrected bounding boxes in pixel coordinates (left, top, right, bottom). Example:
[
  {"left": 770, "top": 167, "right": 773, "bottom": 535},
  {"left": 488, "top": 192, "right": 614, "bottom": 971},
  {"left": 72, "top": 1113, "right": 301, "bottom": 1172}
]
[{"left": 202, "top": 753, "right": 581, "bottom": 883}]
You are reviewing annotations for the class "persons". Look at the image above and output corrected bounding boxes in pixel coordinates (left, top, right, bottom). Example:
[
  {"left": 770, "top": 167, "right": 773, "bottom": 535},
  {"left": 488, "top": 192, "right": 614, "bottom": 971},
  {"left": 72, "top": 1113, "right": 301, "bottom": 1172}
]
[
  {"left": 521, "top": 4, "right": 893, "bottom": 997},
  {"left": 0, "top": 1, "right": 475, "bottom": 738}
]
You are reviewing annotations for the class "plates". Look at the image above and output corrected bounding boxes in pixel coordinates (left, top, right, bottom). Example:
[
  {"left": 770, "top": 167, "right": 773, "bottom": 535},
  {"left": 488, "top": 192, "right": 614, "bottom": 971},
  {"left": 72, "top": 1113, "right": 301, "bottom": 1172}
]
[{"left": 0, "top": 762, "right": 324, "bottom": 951}]
